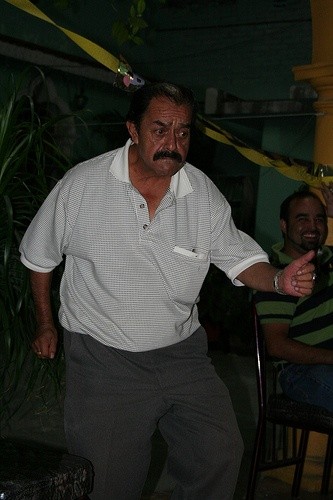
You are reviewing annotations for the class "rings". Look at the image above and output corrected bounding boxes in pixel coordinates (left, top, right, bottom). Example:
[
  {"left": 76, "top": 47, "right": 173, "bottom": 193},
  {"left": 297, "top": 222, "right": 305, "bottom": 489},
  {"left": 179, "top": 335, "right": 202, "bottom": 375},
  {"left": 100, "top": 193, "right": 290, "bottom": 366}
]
[
  {"left": 36, "top": 351, "right": 42, "bottom": 356},
  {"left": 312, "top": 272, "right": 317, "bottom": 280}
]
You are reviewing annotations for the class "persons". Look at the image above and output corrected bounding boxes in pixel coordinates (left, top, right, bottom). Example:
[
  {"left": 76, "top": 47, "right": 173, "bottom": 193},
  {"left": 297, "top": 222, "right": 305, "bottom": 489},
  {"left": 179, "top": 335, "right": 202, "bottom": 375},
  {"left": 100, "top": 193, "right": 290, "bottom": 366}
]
[
  {"left": 19, "top": 80, "right": 316, "bottom": 500},
  {"left": 254, "top": 180, "right": 333, "bottom": 412}
]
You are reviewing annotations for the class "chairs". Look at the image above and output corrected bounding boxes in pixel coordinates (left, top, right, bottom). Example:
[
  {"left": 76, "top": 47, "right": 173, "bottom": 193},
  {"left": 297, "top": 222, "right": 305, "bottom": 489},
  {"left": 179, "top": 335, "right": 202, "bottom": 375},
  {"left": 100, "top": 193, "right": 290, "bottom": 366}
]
[{"left": 246, "top": 292, "right": 333, "bottom": 500}]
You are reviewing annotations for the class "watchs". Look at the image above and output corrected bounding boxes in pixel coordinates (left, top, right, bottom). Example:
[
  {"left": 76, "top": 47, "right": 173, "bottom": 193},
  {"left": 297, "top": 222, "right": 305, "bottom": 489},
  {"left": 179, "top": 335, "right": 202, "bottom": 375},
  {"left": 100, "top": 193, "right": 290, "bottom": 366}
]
[{"left": 272, "top": 269, "right": 288, "bottom": 296}]
